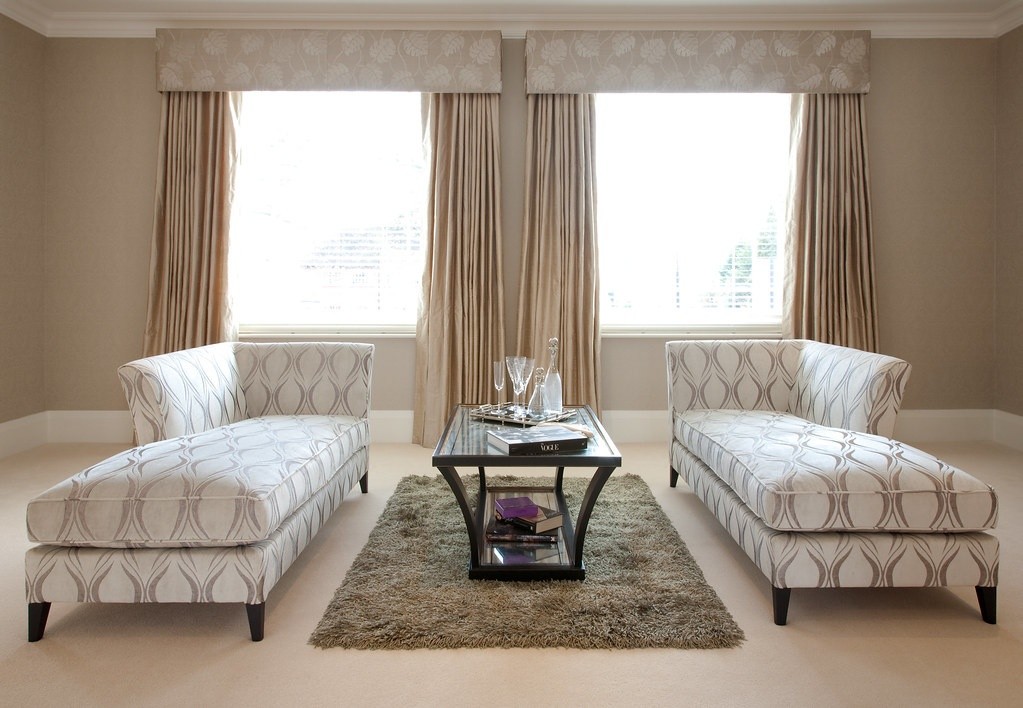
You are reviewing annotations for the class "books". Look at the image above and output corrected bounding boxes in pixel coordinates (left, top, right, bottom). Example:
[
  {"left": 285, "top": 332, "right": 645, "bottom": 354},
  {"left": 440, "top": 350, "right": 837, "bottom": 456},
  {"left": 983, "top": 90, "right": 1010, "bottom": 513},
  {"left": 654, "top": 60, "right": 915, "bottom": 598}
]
[
  {"left": 486, "top": 425, "right": 588, "bottom": 455},
  {"left": 486, "top": 496, "right": 565, "bottom": 543},
  {"left": 486, "top": 543, "right": 559, "bottom": 565}
]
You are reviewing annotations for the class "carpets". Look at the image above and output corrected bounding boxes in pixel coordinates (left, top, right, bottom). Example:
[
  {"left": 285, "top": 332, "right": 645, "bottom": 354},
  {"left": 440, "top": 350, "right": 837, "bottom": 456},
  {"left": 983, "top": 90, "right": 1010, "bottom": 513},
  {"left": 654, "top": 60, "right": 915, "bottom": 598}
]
[{"left": 308, "top": 474, "right": 745, "bottom": 651}]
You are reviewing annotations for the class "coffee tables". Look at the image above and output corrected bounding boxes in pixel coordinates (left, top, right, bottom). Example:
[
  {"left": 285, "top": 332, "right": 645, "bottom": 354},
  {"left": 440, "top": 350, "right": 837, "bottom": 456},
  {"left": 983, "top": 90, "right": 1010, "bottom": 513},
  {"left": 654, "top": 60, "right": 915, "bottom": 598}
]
[{"left": 432, "top": 404, "right": 622, "bottom": 583}]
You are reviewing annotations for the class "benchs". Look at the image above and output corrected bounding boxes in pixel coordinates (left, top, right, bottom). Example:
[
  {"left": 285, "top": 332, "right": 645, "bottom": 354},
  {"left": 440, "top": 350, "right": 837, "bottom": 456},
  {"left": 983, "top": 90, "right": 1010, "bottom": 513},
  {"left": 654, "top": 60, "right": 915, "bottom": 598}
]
[
  {"left": 665, "top": 338, "right": 1000, "bottom": 626},
  {"left": 23, "top": 341, "right": 376, "bottom": 644}
]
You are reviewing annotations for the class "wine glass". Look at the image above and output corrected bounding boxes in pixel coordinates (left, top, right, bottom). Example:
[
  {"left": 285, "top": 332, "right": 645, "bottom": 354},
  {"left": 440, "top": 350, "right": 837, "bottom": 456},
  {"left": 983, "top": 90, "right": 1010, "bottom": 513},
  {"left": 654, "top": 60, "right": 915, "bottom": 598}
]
[{"left": 493, "top": 355, "right": 535, "bottom": 420}]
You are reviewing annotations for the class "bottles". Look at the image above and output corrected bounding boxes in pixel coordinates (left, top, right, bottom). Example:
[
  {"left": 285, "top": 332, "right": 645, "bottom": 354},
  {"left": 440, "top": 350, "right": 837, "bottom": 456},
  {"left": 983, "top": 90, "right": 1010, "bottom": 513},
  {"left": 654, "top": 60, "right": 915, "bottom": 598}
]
[
  {"left": 527, "top": 367, "right": 551, "bottom": 419},
  {"left": 543, "top": 338, "right": 562, "bottom": 414}
]
